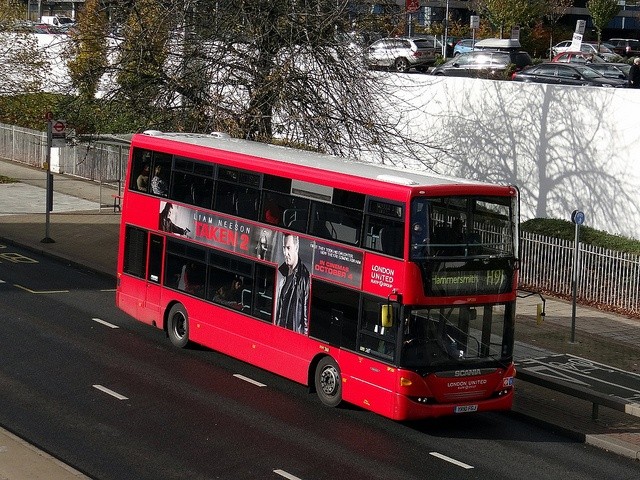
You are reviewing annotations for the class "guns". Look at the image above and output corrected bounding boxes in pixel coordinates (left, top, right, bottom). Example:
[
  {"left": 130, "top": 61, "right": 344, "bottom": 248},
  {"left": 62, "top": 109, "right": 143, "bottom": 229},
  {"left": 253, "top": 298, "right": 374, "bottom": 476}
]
[{"left": 184, "top": 227, "right": 191, "bottom": 233}]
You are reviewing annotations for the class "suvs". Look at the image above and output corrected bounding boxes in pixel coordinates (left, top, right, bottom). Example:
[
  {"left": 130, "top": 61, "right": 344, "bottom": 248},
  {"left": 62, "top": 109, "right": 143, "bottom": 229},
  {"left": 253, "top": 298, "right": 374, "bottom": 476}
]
[
  {"left": 362, "top": 36, "right": 437, "bottom": 73},
  {"left": 430, "top": 38, "right": 533, "bottom": 81}
]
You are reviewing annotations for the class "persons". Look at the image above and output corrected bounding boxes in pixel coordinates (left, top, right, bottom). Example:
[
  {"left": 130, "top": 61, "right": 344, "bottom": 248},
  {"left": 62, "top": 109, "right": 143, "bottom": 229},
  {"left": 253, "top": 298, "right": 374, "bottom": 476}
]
[
  {"left": 152, "top": 165, "right": 167, "bottom": 195},
  {"left": 273, "top": 230, "right": 310, "bottom": 336},
  {"left": 213, "top": 288, "right": 237, "bottom": 306},
  {"left": 229, "top": 277, "right": 243, "bottom": 303},
  {"left": 137, "top": 164, "right": 150, "bottom": 193},
  {"left": 159, "top": 202, "right": 189, "bottom": 236},
  {"left": 428, "top": 331, "right": 466, "bottom": 373}
]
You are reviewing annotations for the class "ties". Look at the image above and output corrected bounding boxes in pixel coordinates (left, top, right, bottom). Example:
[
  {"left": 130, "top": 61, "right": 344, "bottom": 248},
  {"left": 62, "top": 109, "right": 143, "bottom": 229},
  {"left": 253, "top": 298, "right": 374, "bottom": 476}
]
[{"left": 284, "top": 273, "right": 294, "bottom": 301}]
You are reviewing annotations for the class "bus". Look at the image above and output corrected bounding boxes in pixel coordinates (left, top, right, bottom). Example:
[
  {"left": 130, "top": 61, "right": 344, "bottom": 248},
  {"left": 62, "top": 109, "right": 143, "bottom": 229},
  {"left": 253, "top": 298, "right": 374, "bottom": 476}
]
[{"left": 114, "top": 131, "right": 522, "bottom": 421}]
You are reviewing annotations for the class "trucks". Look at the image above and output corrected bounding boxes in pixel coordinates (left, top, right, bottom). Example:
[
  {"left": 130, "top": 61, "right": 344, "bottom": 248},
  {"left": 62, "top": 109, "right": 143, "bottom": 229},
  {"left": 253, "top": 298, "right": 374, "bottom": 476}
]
[{"left": 41, "top": 14, "right": 74, "bottom": 27}]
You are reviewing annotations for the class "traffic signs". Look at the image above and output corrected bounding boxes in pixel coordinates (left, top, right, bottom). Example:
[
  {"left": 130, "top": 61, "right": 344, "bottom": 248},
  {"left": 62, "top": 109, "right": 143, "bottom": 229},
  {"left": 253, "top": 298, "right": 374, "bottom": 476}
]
[
  {"left": 45, "top": 113, "right": 68, "bottom": 136},
  {"left": 571, "top": 210, "right": 585, "bottom": 226}
]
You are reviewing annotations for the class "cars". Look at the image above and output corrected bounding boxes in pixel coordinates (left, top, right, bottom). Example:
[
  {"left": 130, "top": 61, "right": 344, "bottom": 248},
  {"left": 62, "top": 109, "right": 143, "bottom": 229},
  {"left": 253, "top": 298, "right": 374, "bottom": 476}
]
[
  {"left": 512, "top": 63, "right": 634, "bottom": 88},
  {"left": 453, "top": 39, "right": 498, "bottom": 57},
  {"left": 587, "top": 63, "right": 632, "bottom": 79},
  {"left": 551, "top": 52, "right": 606, "bottom": 64},
  {"left": 581, "top": 44, "right": 623, "bottom": 63},
  {"left": 546, "top": 40, "right": 586, "bottom": 58}
]
